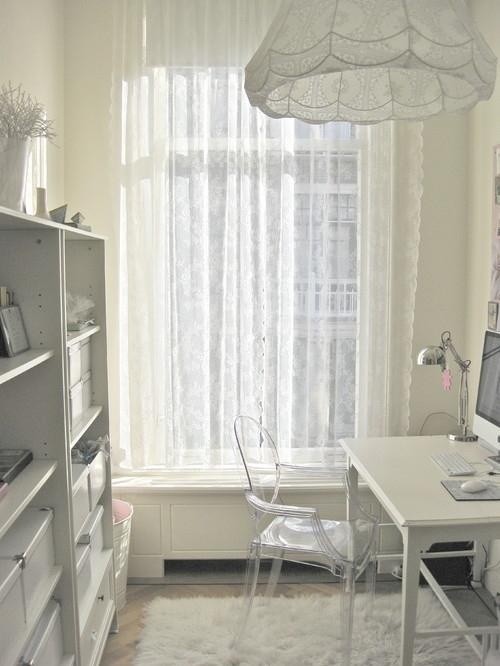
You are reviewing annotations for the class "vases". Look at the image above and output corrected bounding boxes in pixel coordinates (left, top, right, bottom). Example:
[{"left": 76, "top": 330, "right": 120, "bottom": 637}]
[
  {"left": 1, "top": 137, "right": 55, "bottom": 210},
  {"left": 33, "top": 187, "right": 53, "bottom": 223}
]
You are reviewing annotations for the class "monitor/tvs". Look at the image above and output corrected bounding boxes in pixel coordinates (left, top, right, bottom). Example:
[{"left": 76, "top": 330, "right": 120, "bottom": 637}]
[{"left": 474, "top": 329, "right": 500, "bottom": 470}]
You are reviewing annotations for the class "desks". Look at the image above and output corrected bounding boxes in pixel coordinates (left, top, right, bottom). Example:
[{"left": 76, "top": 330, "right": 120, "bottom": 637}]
[{"left": 341, "top": 433, "right": 499, "bottom": 665}]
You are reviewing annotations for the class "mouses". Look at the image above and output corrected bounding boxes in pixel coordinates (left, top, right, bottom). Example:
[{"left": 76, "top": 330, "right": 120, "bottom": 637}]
[{"left": 461, "top": 480, "right": 489, "bottom": 492}]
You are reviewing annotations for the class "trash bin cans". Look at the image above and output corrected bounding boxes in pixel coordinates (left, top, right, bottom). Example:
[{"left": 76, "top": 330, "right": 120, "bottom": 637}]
[{"left": 113, "top": 500, "right": 133, "bottom": 613}]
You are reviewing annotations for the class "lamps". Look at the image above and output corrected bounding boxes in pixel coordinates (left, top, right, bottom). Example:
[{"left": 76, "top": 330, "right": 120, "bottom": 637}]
[
  {"left": 416, "top": 331, "right": 483, "bottom": 442},
  {"left": 242, "top": 1, "right": 500, "bottom": 124}
]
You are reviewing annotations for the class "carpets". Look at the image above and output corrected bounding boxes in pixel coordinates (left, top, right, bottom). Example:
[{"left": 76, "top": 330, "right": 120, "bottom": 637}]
[{"left": 133, "top": 586, "right": 478, "bottom": 666}]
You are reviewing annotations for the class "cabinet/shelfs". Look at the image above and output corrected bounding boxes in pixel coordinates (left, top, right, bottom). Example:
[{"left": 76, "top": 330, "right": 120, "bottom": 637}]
[
  {"left": 63, "top": 224, "right": 117, "bottom": 666},
  {"left": 0, "top": 208, "right": 81, "bottom": 665}
]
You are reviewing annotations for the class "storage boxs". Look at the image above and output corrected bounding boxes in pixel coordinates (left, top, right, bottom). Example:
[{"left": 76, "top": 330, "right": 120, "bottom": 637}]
[
  {"left": 68, "top": 336, "right": 93, "bottom": 434},
  {"left": 75, "top": 504, "right": 105, "bottom": 581},
  {"left": 74, "top": 546, "right": 91, "bottom": 609},
  {"left": 72, "top": 437, "right": 106, "bottom": 540},
  {"left": 0, "top": 558, "right": 26, "bottom": 664},
  {"left": 20, "top": 598, "right": 64, "bottom": 665},
  {"left": 0, "top": 508, "right": 57, "bottom": 623}
]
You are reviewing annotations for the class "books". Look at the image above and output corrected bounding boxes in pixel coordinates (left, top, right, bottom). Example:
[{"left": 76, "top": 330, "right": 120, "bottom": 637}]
[{"left": 20, "top": 108, "right": 49, "bottom": 218}]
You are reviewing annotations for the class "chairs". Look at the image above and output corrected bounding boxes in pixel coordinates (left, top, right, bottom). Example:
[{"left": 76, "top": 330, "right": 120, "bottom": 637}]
[{"left": 235, "top": 413, "right": 378, "bottom": 666}]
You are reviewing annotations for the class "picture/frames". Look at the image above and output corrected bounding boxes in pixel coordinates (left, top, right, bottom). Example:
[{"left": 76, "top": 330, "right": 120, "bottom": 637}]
[{"left": 0, "top": 303, "right": 31, "bottom": 359}]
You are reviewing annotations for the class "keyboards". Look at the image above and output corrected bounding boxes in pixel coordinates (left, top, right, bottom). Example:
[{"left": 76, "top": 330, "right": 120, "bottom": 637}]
[{"left": 430, "top": 452, "right": 475, "bottom": 476}]
